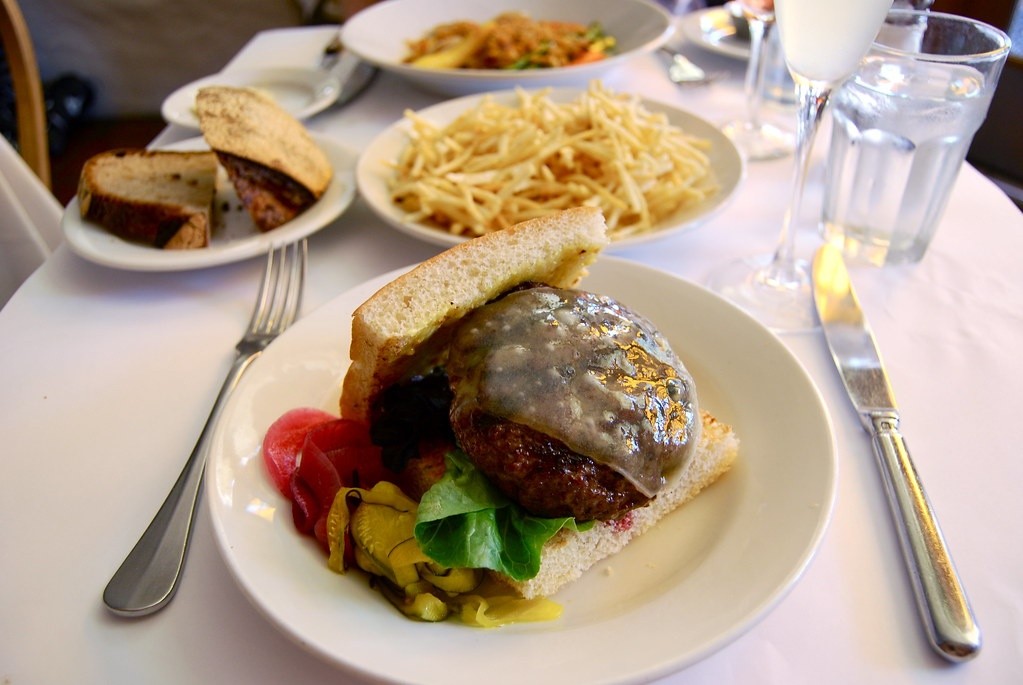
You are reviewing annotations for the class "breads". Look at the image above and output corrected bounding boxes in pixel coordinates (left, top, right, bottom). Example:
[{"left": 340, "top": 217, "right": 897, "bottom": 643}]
[
  {"left": 80, "top": 147, "right": 220, "bottom": 252},
  {"left": 196, "top": 86, "right": 334, "bottom": 232}
]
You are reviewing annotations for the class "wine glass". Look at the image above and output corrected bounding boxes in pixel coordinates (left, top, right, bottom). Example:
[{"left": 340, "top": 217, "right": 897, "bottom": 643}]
[
  {"left": 713, "top": 1, "right": 798, "bottom": 159},
  {"left": 708, "top": 1, "right": 895, "bottom": 335}
]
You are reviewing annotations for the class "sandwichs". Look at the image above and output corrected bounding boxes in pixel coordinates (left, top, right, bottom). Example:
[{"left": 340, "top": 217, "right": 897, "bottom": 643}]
[{"left": 334, "top": 203, "right": 742, "bottom": 601}]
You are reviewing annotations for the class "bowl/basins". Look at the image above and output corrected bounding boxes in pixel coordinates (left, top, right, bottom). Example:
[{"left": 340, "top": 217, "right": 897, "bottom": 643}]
[{"left": 338, "top": 0, "right": 679, "bottom": 100}]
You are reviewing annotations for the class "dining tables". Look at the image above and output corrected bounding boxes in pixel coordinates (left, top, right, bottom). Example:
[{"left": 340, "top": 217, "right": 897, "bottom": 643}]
[{"left": 0, "top": 26, "right": 1023, "bottom": 685}]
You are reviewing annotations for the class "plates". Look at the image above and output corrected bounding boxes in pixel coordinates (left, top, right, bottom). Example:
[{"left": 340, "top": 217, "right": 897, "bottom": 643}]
[
  {"left": 160, "top": 63, "right": 343, "bottom": 133},
  {"left": 681, "top": 5, "right": 766, "bottom": 62},
  {"left": 61, "top": 132, "right": 359, "bottom": 272},
  {"left": 355, "top": 85, "right": 747, "bottom": 255},
  {"left": 203, "top": 257, "right": 839, "bottom": 685}
]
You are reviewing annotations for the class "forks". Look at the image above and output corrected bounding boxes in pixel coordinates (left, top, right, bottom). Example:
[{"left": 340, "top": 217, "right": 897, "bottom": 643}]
[{"left": 101, "top": 237, "right": 309, "bottom": 620}]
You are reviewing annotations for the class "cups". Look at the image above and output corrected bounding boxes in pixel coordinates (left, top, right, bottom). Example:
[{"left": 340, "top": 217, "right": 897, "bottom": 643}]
[{"left": 818, "top": 8, "right": 1011, "bottom": 266}]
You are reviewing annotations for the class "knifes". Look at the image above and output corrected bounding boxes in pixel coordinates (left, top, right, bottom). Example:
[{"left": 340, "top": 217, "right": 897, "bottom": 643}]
[{"left": 811, "top": 240, "right": 985, "bottom": 665}]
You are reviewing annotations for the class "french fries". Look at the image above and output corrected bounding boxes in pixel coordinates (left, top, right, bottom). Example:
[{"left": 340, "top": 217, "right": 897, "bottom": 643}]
[{"left": 383, "top": 80, "right": 722, "bottom": 240}]
